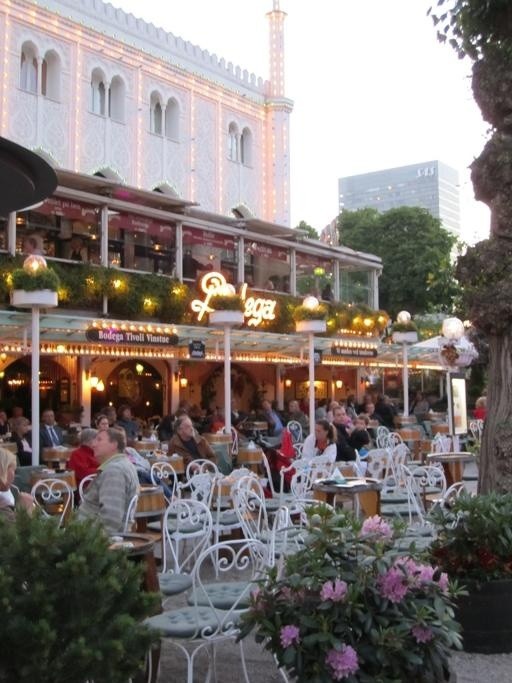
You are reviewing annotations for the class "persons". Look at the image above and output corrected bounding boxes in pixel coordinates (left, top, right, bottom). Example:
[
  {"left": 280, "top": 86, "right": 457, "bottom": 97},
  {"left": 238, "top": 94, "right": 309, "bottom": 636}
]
[
  {"left": 182, "top": 254, "right": 208, "bottom": 279},
  {"left": 245, "top": 271, "right": 293, "bottom": 295},
  {"left": 69, "top": 236, "right": 88, "bottom": 261},
  {"left": 1, "top": 389, "right": 487, "bottom": 532},
  {"left": 22, "top": 238, "right": 44, "bottom": 255},
  {"left": 29, "top": 227, "right": 50, "bottom": 250},
  {"left": 321, "top": 283, "right": 334, "bottom": 300}
]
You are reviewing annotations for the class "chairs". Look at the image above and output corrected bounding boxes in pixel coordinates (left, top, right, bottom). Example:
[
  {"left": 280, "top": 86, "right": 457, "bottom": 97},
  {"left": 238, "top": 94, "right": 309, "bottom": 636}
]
[{"left": 1, "top": 410, "right": 483, "bottom": 682}]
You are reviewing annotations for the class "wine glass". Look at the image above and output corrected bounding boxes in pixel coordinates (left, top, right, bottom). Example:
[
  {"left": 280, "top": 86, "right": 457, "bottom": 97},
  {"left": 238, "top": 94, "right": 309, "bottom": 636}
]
[{"left": 161, "top": 441, "right": 169, "bottom": 460}]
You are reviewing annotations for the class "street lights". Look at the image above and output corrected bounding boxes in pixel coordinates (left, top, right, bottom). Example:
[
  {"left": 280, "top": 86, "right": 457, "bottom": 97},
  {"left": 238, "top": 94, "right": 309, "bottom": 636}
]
[
  {"left": 440, "top": 316, "right": 467, "bottom": 451},
  {"left": 12, "top": 253, "right": 61, "bottom": 468},
  {"left": 391, "top": 309, "right": 419, "bottom": 414},
  {"left": 209, "top": 283, "right": 246, "bottom": 435},
  {"left": 295, "top": 294, "right": 327, "bottom": 432}
]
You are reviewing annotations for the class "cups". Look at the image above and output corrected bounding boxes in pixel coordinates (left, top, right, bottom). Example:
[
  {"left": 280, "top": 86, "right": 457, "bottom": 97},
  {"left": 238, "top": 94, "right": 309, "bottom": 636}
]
[
  {"left": 137, "top": 431, "right": 143, "bottom": 440},
  {"left": 353, "top": 461, "right": 367, "bottom": 480}
]
